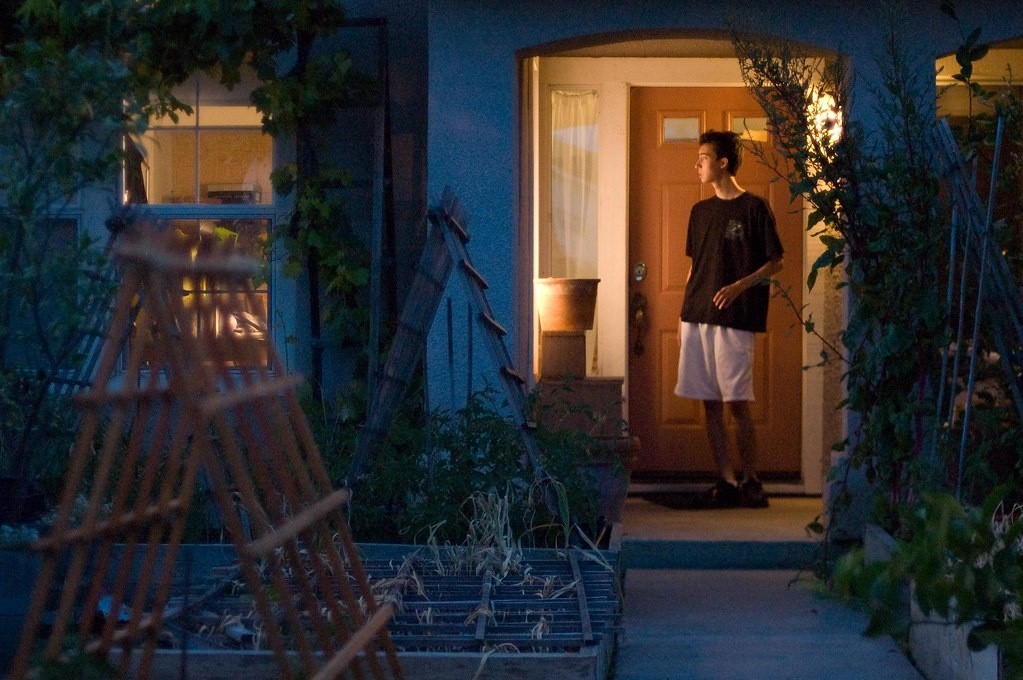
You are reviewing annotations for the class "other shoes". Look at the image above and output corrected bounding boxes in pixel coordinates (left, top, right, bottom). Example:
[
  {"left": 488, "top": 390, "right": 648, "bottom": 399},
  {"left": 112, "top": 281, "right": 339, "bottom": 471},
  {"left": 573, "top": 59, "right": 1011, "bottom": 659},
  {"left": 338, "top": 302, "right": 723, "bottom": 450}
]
[
  {"left": 738, "top": 478, "right": 769, "bottom": 505},
  {"left": 707, "top": 477, "right": 736, "bottom": 507}
]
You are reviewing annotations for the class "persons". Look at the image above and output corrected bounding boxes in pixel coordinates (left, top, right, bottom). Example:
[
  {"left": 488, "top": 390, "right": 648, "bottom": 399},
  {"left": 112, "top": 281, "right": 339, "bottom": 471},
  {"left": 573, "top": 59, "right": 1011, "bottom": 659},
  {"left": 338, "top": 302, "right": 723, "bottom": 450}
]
[{"left": 674, "top": 130, "right": 786, "bottom": 507}]
